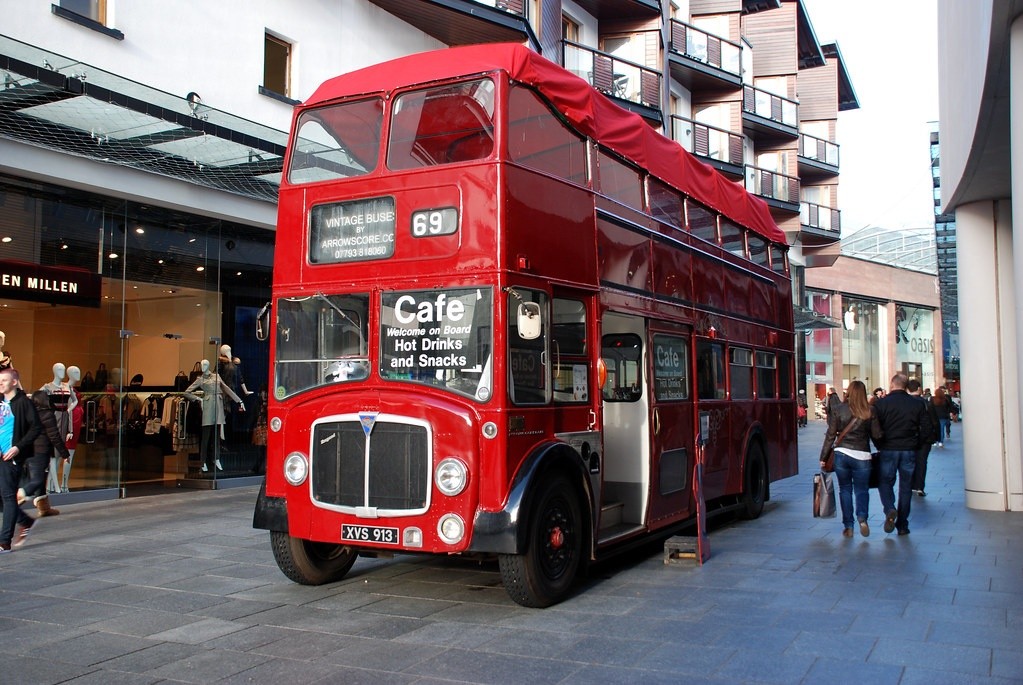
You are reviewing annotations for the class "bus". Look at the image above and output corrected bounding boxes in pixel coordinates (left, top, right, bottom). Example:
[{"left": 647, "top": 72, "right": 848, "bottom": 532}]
[{"left": 249, "top": 41, "right": 800, "bottom": 609}]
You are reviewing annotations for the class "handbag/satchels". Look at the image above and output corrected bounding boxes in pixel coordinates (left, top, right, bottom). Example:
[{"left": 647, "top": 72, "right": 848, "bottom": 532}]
[
  {"left": 175, "top": 371, "right": 188, "bottom": 386},
  {"left": 95, "top": 363, "right": 107, "bottom": 387},
  {"left": 81, "top": 372, "right": 94, "bottom": 389},
  {"left": 822, "top": 450, "right": 836, "bottom": 472},
  {"left": 867, "top": 452, "right": 895, "bottom": 488},
  {"left": 190, "top": 362, "right": 205, "bottom": 386},
  {"left": 812, "top": 470, "right": 839, "bottom": 519}
]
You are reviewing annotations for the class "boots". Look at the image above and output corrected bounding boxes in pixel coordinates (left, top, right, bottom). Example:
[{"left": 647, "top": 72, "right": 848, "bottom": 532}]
[
  {"left": 16, "top": 488, "right": 32, "bottom": 507},
  {"left": 35, "top": 493, "right": 60, "bottom": 516}
]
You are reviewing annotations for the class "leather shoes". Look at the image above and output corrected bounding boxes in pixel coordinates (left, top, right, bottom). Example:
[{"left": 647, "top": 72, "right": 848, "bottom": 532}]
[
  {"left": 843, "top": 528, "right": 853, "bottom": 538},
  {"left": 860, "top": 517, "right": 870, "bottom": 538}
]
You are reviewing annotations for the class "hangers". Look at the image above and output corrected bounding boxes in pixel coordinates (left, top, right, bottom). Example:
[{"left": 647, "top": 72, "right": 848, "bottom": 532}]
[{"left": 81, "top": 394, "right": 188, "bottom": 404}]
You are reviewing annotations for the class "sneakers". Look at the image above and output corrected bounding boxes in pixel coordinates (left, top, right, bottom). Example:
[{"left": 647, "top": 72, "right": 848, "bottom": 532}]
[
  {"left": 14, "top": 518, "right": 37, "bottom": 547},
  {"left": 0, "top": 541, "right": 12, "bottom": 555}
]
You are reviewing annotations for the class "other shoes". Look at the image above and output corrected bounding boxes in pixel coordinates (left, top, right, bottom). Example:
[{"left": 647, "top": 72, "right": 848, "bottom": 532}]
[
  {"left": 883, "top": 507, "right": 896, "bottom": 535},
  {"left": 898, "top": 527, "right": 910, "bottom": 536}
]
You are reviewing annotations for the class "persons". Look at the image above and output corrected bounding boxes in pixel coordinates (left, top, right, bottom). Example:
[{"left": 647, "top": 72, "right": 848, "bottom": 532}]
[
  {"left": 905, "top": 380, "right": 942, "bottom": 497},
  {"left": 184, "top": 360, "right": 246, "bottom": 472},
  {"left": 823, "top": 386, "right": 842, "bottom": 434},
  {"left": 16, "top": 390, "right": 71, "bottom": 518},
  {"left": 95, "top": 363, "right": 108, "bottom": 391},
  {"left": 61, "top": 365, "right": 83, "bottom": 493},
  {"left": 38, "top": 362, "right": 78, "bottom": 495},
  {"left": 920, "top": 386, "right": 962, "bottom": 448},
  {"left": 797, "top": 392, "right": 808, "bottom": 428},
  {"left": 0, "top": 369, "right": 43, "bottom": 555},
  {"left": 0, "top": 331, "right": 24, "bottom": 392},
  {"left": 81, "top": 370, "right": 94, "bottom": 391},
  {"left": 213, "top": 344, "right": 255, "bottom": 451},
  {"left": 819, "top": 381, "right": 884, "bottom": 538},
  {"left": 869, "top": 388, "right": 886, "bottom": 404},
  {"left": 868, "top": 373, "right": 929, "bottom": 535}
]
